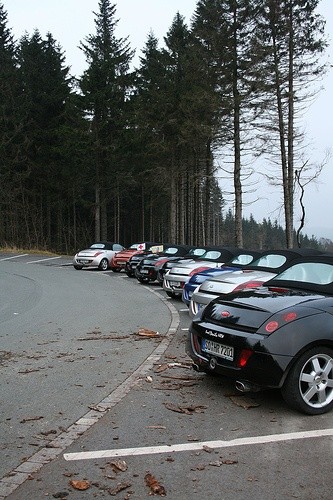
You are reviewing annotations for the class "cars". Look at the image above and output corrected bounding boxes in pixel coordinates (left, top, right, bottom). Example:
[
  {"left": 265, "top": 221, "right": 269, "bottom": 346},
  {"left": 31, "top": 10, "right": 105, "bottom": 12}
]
[
  {"left": 125, "top": 244, "right": 180, "bottom": 278},
  {"left": 183, "top": 251, "right": 261, "bottom": 309},
  {"left": 188, "top": 250, "right": 309, "bottom": 321},
  {"left": 163, "top": 247, "right": 236, "bottom": 297},
  {"left": 136, "top": 246, "right": 196, "bottom": 286},
  {"left": 110, "top": 242, "right": 161, "bottom": 273},
  {"left": 185, "top": 257, "right": 333, "bottom": 416},
  {"left": 73, "top": 242, "right": 127, "bottom": 271}
]
